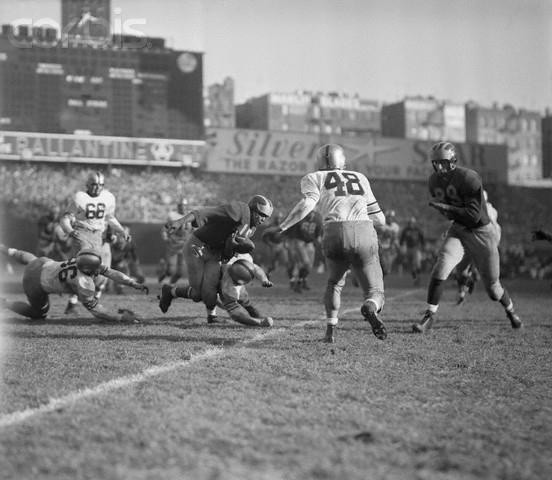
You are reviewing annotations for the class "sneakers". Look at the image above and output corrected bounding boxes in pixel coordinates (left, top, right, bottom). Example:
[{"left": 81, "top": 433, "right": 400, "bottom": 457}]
[
  {"left": 160, "top": 284, "right": 172, "bottom": 313},
  {"left": 412, "top": 312, "right": 433, "bottom": 332},
  {"left": 289, "top": 279, "right": 311, "bottom": 294},
  {"left": 506, "top": 309, "right": 521, "bottom": 328},
  {"left": 361, "top": 304, "right": 387, "bottom": 340},
  {"left": 325, "top": 325, "right": 335, "bottom": 342}
]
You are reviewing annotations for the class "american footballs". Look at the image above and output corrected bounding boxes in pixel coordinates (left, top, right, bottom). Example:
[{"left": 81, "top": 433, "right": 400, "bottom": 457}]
[{"left": 233, "top": 237, "right": 254, "bottom": 253}]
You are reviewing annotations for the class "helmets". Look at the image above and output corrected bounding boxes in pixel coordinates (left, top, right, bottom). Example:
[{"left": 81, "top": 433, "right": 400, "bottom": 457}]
[
  {"left": 429, "top": 142, "right": 456, "bottom": 161},
  {"left": 248, "top": 195, "right": 273, "bottom": 217},
  {"left": 316, "top": 144, "right": 346, "bottom": 170},
  {"left": 77, "top": 249, "right": 102, "bottom": 275},
  {"left": 86, "top": 172, "right": 104, "bottom": 184},
  {"left": 231, "top": 259, "right": 255, "bottom": 285}
]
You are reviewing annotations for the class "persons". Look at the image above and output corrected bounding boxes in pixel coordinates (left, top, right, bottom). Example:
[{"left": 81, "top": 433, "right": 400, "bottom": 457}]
[
  {"left": 410, "top": 140, "right": 551, "bottom": 334},
  {"left": 261, "top": 202, "right": 425, "bottom": 292},
  {"left": 261, "top": 143, "right": 387, "bottom": 342},
  {"left": 3, "top": 169, "right": 149, "bottom": 324},
  {"left": 156, "top": 195, "right": 274, "bottom": 326}
]
